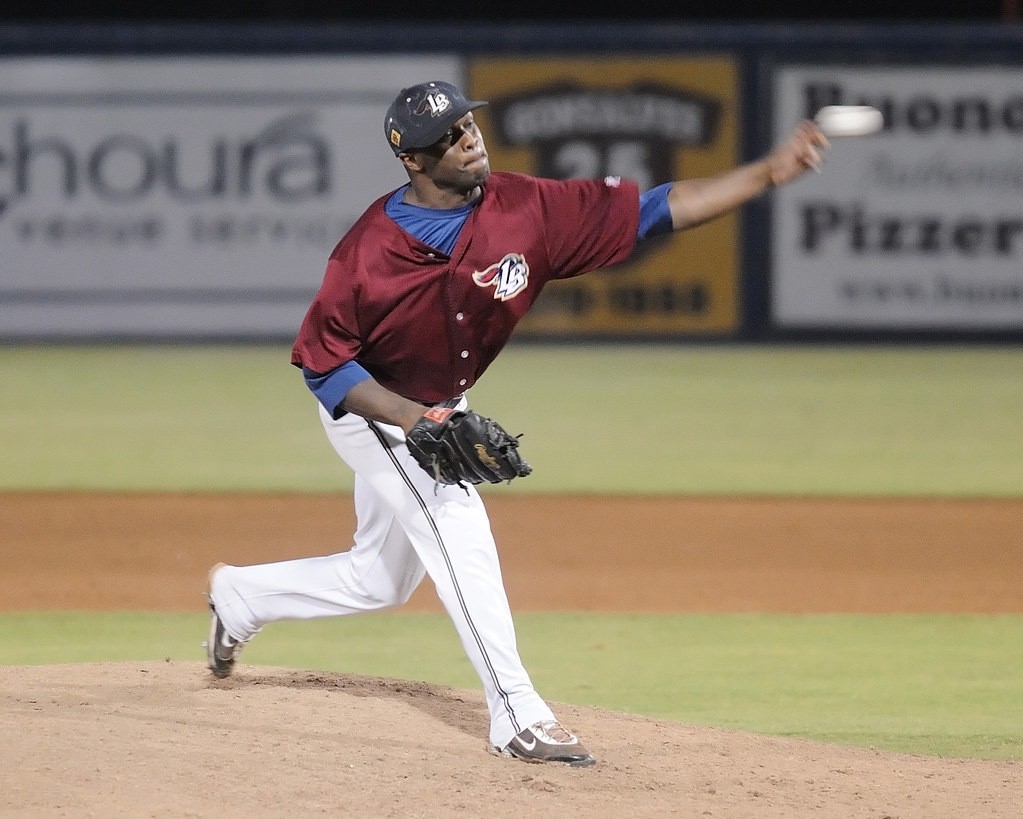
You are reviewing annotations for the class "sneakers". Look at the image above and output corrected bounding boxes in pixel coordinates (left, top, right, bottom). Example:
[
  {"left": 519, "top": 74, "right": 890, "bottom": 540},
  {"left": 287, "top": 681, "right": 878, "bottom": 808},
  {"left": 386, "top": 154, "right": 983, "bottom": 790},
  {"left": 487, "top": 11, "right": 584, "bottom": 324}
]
[
  {"left": 485, "top": 715, "right": 597, "bottom": 768},
  {"left": 201, "top": 562, "right": 245, "bottom": 679}
]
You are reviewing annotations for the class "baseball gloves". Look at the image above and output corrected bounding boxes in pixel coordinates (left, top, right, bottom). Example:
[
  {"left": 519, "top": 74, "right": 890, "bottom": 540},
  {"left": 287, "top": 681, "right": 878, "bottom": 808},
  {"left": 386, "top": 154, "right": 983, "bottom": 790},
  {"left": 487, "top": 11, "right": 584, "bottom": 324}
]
[{"left": 405, "top": 408, "right": 534, "bottom": 487}]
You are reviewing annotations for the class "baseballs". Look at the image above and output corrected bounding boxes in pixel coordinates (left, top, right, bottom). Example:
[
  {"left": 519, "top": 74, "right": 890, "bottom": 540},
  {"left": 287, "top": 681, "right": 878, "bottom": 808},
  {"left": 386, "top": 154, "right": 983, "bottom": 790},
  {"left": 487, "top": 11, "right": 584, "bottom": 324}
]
[{"left": 818, "top": 102, "right": 882, "bottom": 136}]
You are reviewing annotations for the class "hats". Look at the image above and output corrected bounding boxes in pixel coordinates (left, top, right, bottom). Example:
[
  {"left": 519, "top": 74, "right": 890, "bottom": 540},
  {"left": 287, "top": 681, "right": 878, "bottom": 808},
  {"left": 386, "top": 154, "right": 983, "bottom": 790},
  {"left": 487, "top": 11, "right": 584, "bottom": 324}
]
[{"left": 384, "top": 80, "right": 489, "bottom": 158}]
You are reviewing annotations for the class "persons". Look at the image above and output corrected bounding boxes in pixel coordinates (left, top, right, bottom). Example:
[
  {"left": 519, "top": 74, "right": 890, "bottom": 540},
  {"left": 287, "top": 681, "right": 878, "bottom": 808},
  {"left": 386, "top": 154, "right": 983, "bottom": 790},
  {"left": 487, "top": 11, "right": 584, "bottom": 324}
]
[{"left": 204, "top": 81, "right": 829, "bottom": 766}]
